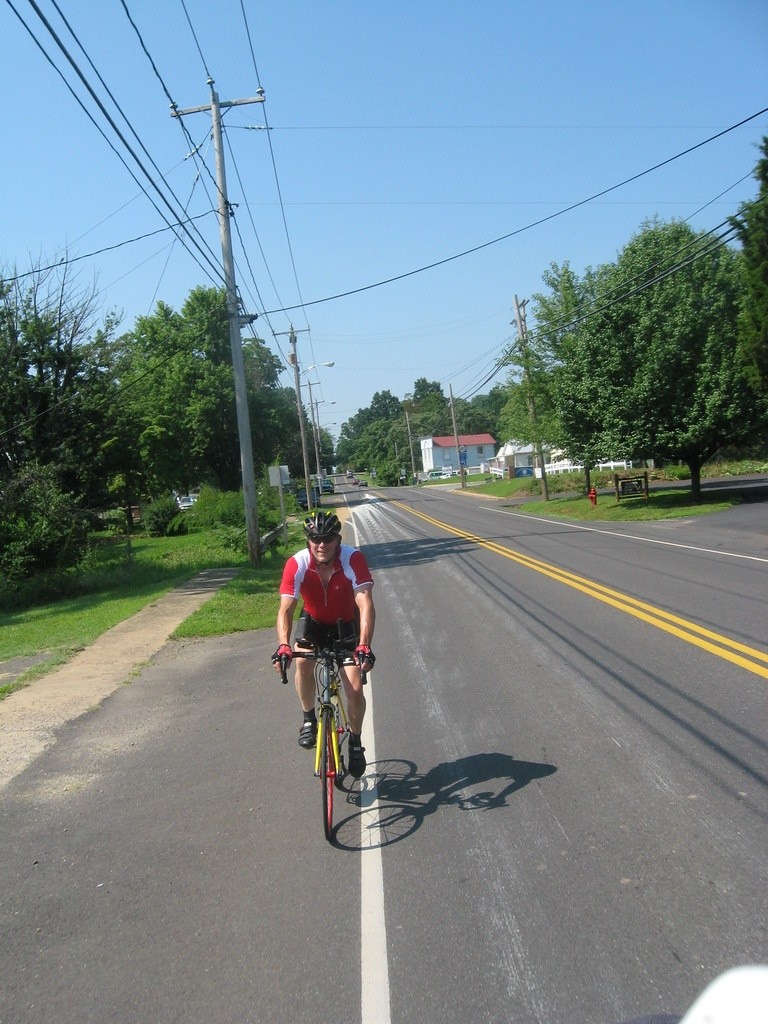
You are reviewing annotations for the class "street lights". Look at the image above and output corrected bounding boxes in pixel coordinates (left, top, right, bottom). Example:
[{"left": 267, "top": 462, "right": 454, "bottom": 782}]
[{"left": 293, "top": 360, "right": 336, "bottom": 513}]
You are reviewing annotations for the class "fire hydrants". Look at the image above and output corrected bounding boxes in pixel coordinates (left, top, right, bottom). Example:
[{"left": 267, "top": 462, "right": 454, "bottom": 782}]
[{"left": 588, "top": 488, "right": 598, "bottom": 506}]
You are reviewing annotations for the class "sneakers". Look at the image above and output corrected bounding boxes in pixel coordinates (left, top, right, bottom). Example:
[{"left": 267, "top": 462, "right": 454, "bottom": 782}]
[
  {"left": 348, "top": 738, "right": 367, "bottom": 779},
  {"left": 298, "top": 717, "right": 318, "bottom": 747}
]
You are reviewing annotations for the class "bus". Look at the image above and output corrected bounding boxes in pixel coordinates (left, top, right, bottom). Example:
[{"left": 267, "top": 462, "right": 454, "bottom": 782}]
[{"left": 347, "top": 469, "right": 353, "bottom": 478}]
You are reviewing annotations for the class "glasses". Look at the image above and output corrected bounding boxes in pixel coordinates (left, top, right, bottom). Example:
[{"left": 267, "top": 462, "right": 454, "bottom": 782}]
[{"left": 309, "top": 534, "right": 338, "bottom": 544}]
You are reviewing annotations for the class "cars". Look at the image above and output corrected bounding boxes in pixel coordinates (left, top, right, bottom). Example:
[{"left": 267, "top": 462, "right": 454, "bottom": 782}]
[
  {"left": 177, "top": 494, "right": 199, "bottom": 512},
  {"left": 358, "top": 479, "right": 368, "bottom": 487},
  {"left": 314, "top": 478, "right": 334, "bottom": 495},
  {"left": 295, "top": 488, "right": 320, "bottom": 509},
  {"left": 352, "top": 477, "right": 360, "bottom": 486},
  {"left": 428, "top": 470, "right": 468, "bottom": 481}
]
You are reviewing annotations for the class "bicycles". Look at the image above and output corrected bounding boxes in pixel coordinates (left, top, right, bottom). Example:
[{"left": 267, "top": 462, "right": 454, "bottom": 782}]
[{"left": 276, "top": 647, "right": 368, "bottom": 841}]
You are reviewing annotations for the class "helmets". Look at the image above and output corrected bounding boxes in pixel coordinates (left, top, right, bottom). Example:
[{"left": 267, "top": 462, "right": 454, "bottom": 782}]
[{"left": 303, "top": 510, "right": 342, "bottom": 538}]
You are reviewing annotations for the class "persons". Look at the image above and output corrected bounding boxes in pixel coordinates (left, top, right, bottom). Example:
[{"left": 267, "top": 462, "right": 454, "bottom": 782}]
[{"left": 271, "top": 510, "right": 376, "bottom": 777}]
